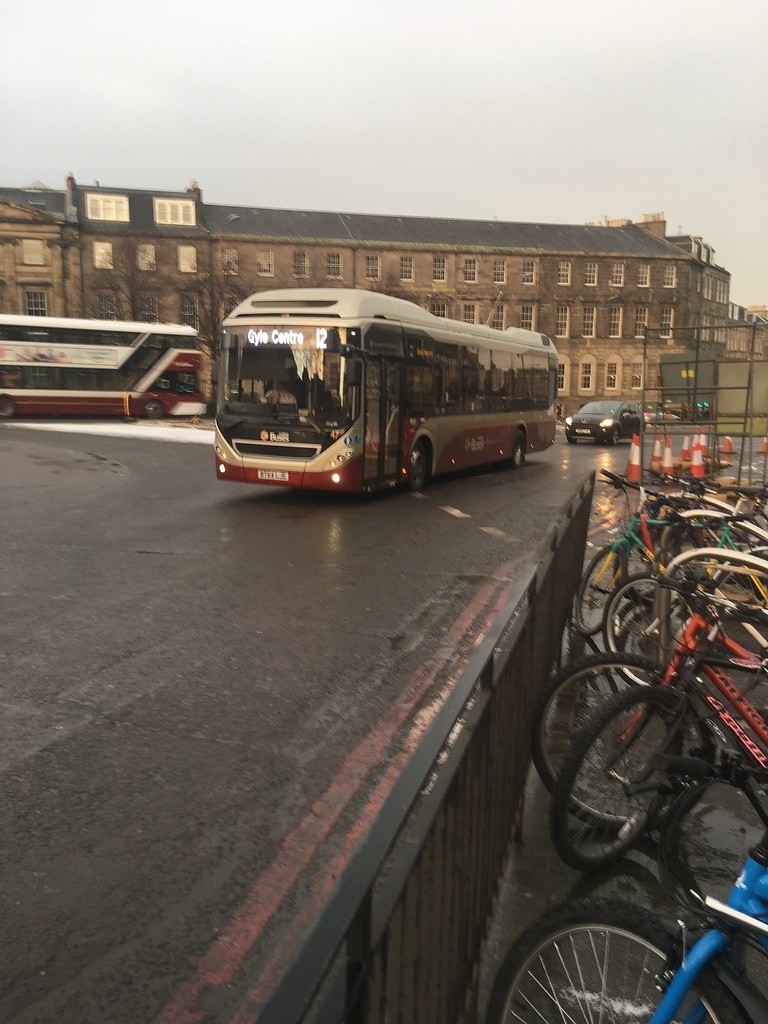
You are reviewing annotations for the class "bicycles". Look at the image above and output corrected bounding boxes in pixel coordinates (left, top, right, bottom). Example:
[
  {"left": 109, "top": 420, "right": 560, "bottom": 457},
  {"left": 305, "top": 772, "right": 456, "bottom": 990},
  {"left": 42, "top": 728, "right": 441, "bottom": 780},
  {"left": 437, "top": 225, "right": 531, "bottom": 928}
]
[{"left": 483, "top": 469, "right": 767, "bottom": 1024}]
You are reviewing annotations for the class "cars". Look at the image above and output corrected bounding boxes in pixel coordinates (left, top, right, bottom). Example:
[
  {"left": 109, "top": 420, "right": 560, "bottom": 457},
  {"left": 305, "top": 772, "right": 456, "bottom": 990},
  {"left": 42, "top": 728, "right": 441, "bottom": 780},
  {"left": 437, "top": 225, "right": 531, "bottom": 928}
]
[
  {"left": 564, "top": 400, "right": 645, "bottom": 444},
  {"left": 642, "top": 406, "right": 681, "bottom": 426}
]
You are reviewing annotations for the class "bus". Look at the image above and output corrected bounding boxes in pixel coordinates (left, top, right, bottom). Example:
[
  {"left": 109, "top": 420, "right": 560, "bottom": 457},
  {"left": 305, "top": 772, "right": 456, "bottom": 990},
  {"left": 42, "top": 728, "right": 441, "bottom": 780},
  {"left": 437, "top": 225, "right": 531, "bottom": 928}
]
[{"left": 0, "top": 313, "right": 208, "bottom": 419}]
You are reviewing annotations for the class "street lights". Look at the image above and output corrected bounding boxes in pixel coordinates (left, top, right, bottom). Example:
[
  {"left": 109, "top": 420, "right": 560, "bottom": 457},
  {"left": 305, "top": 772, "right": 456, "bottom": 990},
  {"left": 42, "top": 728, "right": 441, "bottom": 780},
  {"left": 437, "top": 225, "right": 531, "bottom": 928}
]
[
  {"left": 213, "top": 288, "right": 559, "bottom": 496},
  {"left": 209, "top": 213, "right": 240, "bottom": 352}
]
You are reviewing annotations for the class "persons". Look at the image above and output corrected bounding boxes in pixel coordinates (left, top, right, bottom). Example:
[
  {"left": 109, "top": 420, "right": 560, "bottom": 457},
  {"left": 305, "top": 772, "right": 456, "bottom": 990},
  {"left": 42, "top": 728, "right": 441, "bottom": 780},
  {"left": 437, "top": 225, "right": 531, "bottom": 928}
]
[{"left": 261, "top": 376, "right": 297, "bottom": 410}]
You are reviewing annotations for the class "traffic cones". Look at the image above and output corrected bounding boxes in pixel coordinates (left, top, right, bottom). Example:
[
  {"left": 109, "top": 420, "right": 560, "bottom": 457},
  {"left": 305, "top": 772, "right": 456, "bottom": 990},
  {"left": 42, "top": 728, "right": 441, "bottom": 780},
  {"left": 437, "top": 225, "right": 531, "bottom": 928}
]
[
  {"left": 625, "top": 434, "right": 638, "bottom": 473},
  {"left": 722, "top": 433, "right": 734, "bottom": 467},
  {"left": 626, "top": 438, "right": 642, "bottom": 485},
  {"left": 650, "top": 432, "right": 663, "bottom": 474},
  {"left": 760, "top": 437, "right": 768, "bottom": 455},
  {"left": 659, "top": 432, "right": 675, "bottom": 477},
  {"left": 690, "top": 433, "right": 706, "bottom": 480},
  {"left": 680, "top": 427, "right": 710, "bottom": 462}
]
